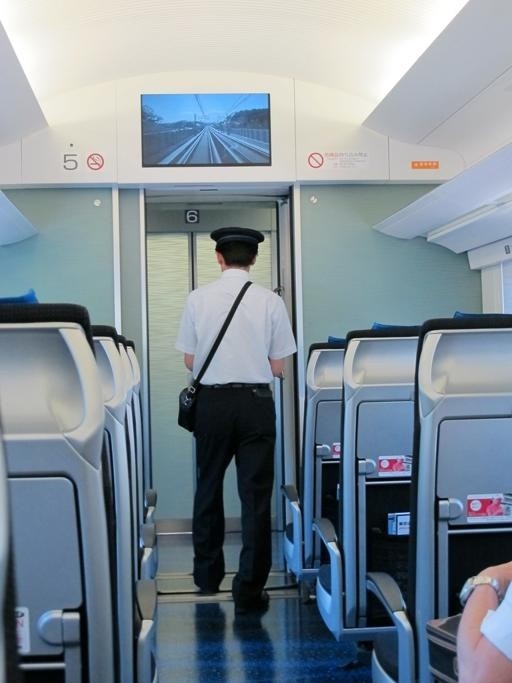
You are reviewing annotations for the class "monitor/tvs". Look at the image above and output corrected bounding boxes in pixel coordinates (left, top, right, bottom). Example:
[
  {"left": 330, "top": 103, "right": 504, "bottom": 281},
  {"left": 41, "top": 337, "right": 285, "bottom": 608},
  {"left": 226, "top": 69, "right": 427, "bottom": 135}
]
[{"left": 141, "top": 93, "right": 273, "bottom": 167}]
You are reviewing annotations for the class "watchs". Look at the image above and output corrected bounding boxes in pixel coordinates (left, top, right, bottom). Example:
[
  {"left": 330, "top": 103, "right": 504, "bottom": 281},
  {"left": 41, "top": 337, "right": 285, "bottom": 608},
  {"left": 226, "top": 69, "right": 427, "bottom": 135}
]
[{"left": 459, "top": 575, "right": 503, "bottom": 606}]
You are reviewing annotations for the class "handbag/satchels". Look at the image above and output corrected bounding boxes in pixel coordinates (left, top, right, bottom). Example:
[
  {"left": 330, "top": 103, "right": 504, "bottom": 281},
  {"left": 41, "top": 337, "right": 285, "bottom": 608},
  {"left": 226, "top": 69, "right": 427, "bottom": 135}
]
[{"left": 178, "top": 386, "right": 196, "bottom": 432}]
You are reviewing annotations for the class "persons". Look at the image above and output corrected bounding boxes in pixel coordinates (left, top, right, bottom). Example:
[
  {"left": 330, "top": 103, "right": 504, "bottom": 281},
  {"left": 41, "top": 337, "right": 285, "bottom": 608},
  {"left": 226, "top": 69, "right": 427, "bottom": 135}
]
[
  {"left": 171, "top": 224, "right": 300, "bottom": 621},
  {"left": 453, "top": 555, "right": 511, "bottom": 681}
]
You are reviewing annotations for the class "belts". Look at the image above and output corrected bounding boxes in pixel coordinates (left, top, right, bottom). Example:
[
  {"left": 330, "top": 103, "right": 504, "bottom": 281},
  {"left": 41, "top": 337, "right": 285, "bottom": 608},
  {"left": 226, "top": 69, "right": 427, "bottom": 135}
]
[{"left": 214, "top": 384, "right": 267, "bottom": 388}]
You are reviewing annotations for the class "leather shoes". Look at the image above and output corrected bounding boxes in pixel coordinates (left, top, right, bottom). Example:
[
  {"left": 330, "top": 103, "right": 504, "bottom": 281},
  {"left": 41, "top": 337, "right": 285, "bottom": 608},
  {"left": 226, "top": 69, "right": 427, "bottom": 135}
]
[{"left": 235, "top": 590, "right": 268, "bottom": 612}]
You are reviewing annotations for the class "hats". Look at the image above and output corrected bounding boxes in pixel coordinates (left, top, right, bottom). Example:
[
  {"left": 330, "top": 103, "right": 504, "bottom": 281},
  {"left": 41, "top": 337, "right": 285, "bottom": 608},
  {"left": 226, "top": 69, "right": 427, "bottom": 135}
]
[{"left": 211, "top": 227, "right": 264, "bottom": 243}]
